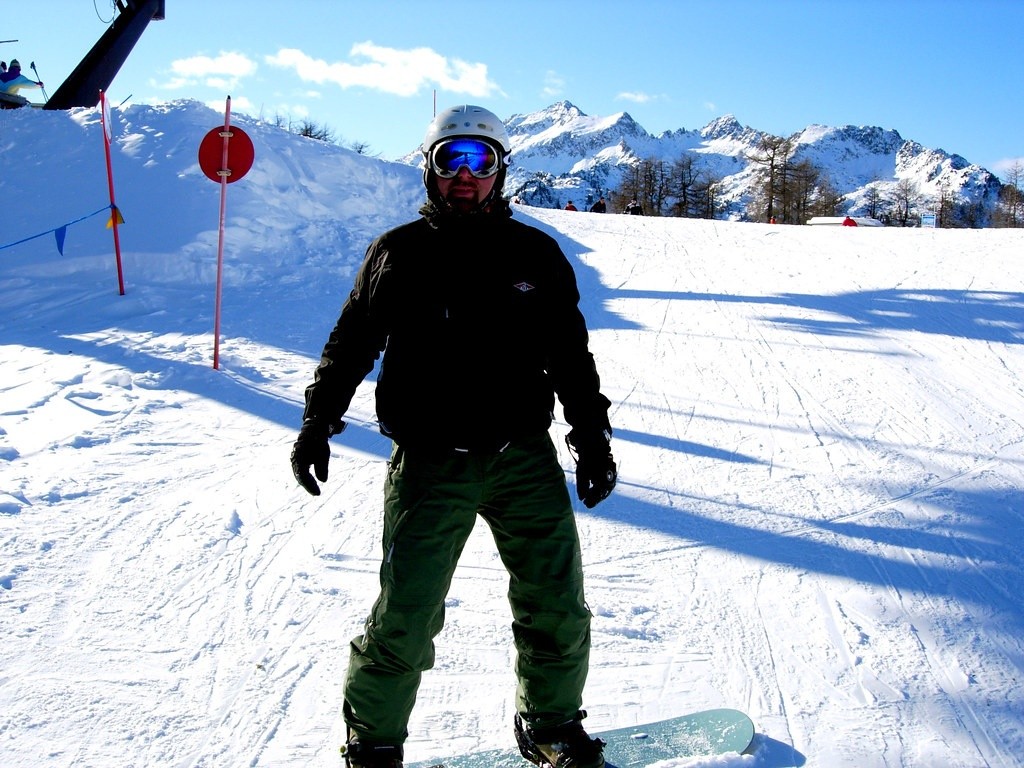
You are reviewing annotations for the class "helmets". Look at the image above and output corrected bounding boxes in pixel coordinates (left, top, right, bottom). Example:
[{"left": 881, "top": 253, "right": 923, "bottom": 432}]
[{"left": 418, "top": 103, "right": 513, "bottom": 221}]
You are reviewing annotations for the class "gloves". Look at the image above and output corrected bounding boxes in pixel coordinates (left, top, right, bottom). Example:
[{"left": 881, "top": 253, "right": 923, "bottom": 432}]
[
  {"left": 564, "top": 418, "right": 619, "bottom": 508},
  {"left": 290, "top": 419, "right": 349, "bottom": 497}
]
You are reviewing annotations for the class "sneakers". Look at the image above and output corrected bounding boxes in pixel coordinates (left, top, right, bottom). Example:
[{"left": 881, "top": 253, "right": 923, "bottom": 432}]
[
  {"left": 340, "top": 725, "right": 409, "bottom": 768},
  {"left": 513, "top": 710, "right": 619, "bottom": 768}
]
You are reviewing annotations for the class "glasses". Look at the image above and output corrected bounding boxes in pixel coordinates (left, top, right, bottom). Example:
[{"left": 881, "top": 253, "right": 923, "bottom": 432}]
[{"left": 426, "top": 138, "right": 501, "bottom": 179}]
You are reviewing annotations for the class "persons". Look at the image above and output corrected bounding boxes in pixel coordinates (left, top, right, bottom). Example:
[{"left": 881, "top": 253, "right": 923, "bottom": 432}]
[
  {"left": 564, "top": 200, "right": 579, "bottom": 211},
  {"left": 770, "top": 215, "right": 777, "bottom": 224},
  {"left": 290, "top": 102, "right": 620, "bottom": 768},
  {"left": 590, "top": 197, "right": 606, "bottom": 213},
  {"left": 624, "top": 198, "right": 644, "bottom": 216},
  {"left": 843, "top": 215, "right": 857, "bottom": 226},
  {"left": 0, "top": 57, "right": 44, "bottom": 96}
]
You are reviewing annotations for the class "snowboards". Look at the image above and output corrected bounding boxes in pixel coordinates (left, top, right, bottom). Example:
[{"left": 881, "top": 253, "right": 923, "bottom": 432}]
[{"left": 404, "top": 708, "right": 756, "bottom": 768}]
